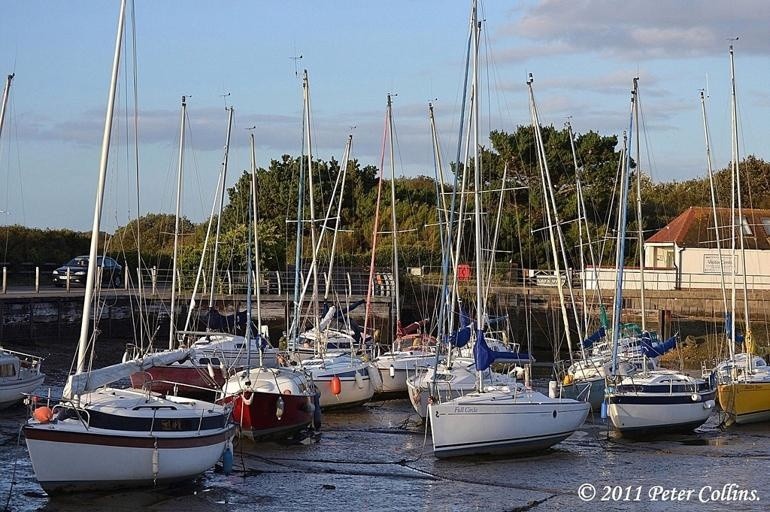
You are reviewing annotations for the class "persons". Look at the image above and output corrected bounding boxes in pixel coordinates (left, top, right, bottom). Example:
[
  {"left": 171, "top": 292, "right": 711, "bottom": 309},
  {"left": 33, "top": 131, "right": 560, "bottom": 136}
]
[
  {"left": 374, "top": 326, "right": 384, "bottom": 344},
  {"left": 412, "top": 336, "right": 423, "bottom": 350},
  {"left": 279, "top": 331, "right": 287, "bottom": 349}
]
